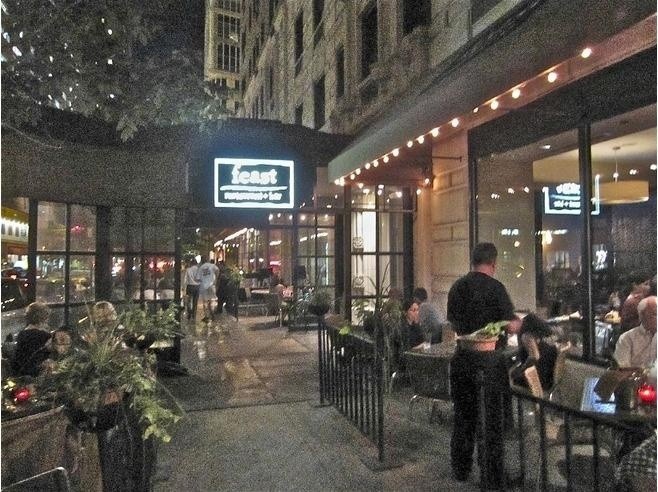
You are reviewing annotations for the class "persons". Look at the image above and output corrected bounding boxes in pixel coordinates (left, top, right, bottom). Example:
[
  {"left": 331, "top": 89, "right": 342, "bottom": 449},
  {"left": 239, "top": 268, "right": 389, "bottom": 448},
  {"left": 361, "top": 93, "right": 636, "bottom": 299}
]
[
  {"left": 14, "top": 298, "right": 54, "bottom": 377},
  {"left": 505, "top": 310, "right": 557, "bottom": 389},
  {"left": 184, "top": 258, "right": 200, "bottom": 320},
  {"left": 612, "top": 294, "right": 656, "bottom": 382},
  {"left": 609, "top": 430, "right": 657, "bottom": 491},
  {"left": 91, "top": 299, "right": 157, "bottom": 357},
  {"left": 194, "top": 253, "right": 221, "bottom": 321},
  {"left": 269, "top": 273, "right": 289, "bottom": 309},
  {"left": 36, "top": 323, "right": 90, "bottom": 372},
  {"left": 445, "top": 242, "right": 524, "bottom": 491},
  {"left": 373, "top": 289, "right": 403, "bottom": 351},
  {"left": 412, "top": 286, "right": 443, "bottom": 344},
  {"left": 396, "top": 295, "right": 427, "bottom": 373},
  {"left": 619, "top": 265, "right": 654, "bottom": 332}
]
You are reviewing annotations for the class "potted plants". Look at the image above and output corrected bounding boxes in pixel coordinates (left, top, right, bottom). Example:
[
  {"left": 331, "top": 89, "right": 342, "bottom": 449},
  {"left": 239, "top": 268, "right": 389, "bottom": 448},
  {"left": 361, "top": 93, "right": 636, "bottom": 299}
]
[
  {"left": 457, "top": 320, "right": 511, "bottom": 352},
  {"left": 18, "top": 287, "right": 193, "bottom": 487},
  {"left": 226, "top": 263, "right": 243, "bottom": 320},
  {"left": 276, "top": 282, "right": 334, "bottom": 325}
]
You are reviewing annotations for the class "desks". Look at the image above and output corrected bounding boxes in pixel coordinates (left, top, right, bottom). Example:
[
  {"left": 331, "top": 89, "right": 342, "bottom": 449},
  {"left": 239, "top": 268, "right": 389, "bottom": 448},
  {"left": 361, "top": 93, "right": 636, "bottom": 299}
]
[{"left": 510, "top": 368, "right": 657, "bottom": 492}]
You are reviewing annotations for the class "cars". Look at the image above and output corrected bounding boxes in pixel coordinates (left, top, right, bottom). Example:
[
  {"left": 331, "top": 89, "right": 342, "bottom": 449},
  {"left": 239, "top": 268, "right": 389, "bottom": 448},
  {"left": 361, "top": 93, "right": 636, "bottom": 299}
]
[{"left": 1, "top": 266, "right": 90, "bottom": 312}]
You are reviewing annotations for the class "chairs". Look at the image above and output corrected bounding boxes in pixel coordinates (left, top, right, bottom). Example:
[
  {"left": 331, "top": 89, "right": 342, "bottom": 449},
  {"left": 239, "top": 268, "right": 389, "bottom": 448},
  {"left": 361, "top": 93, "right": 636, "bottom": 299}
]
[{"left": 385, "top": 330, "right": 572, "bottom": 436}]
